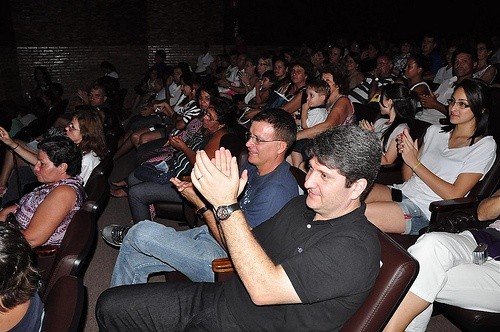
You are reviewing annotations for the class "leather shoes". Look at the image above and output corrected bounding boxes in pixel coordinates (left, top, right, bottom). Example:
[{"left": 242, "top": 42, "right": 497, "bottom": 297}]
[
  {"left": 111, "top": 177, "right": 128, "bottom": 186},
  {"left": 110, "top": 188, "right": 128, "bottom": 197}
]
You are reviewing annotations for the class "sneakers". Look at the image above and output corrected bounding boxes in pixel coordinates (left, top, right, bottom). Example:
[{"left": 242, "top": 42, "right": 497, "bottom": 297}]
[{"left": 101, "top": 223, "right": 133, "bottom": 246}]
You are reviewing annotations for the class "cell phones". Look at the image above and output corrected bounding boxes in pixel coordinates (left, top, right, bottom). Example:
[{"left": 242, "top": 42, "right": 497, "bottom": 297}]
[{"left": 391, "top": 188, "right": 402, "bottom": 202}]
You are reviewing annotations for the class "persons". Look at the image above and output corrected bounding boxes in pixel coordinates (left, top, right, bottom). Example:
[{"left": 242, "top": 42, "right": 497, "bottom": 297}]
[
  {"left": 0, "top": 54, "right": 128, "bottom": 332},
  {"left": 95, "top": 20, "right": 500, "bottom": 332}
]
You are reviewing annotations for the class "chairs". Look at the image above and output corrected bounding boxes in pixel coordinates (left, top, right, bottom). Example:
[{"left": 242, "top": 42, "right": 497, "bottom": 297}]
[
  {"left": 138, "top": 113, "right": 500, "bottom": 332},
  {"left": 0, "top": 87, "right": 128, "bottom": 332}
]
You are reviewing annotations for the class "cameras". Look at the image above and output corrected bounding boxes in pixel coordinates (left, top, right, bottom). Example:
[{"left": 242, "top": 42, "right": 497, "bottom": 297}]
[{"left": 472, "top": 243, "right": 488, "bottom": 264}]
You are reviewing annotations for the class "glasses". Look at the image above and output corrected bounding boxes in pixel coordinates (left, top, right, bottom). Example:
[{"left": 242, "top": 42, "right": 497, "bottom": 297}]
[
  {"left": 68, "top": 121, "right": 81, "bottom": 131},
  {"left": 257, "top": 62, "right": 266, "bottom": 66},
  {"left": 447, "top": 98, "right": 469, "bottom": 108},
  {"left": 246, "top": 132, "right": 287, "bottom": 142}
]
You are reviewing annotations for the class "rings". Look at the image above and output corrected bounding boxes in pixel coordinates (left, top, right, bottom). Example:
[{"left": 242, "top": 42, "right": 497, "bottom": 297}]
[{"left": 197, "top": 176, "right": 203, "bottom": 180}]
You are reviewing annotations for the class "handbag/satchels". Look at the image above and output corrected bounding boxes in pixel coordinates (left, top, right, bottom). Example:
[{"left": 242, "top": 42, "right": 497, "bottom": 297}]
[
  {"left": 126, "top": 114, "right": 164, "bottom": 130},
  {"left": 134, "top": 161, "right": 172, "bottom": 183}
]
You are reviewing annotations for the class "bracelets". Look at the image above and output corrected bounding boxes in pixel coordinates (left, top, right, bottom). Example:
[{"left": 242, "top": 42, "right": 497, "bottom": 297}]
[
  {"left": 412, "top": 162, "right": 422, "bottom": 170},
  {"left": 228, "top": 85, "right": 232, "bottom": 89},
  {"left": 10, "top": 144, "right": 20, "bottom": 152},
  {"left": 246, "top": 84, "right": 251, "bottom": 88}
]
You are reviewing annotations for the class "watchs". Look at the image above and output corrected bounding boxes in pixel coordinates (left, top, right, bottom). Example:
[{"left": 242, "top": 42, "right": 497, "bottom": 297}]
[
  {"left": 374, "top": 77, "right": 380, "bottom": 82},
  {"left": 196, "top": 206, "right": 211, "bottom": 220},
  {"left": 215, "top": 202, "right": 241, "bottom": 225}
]
